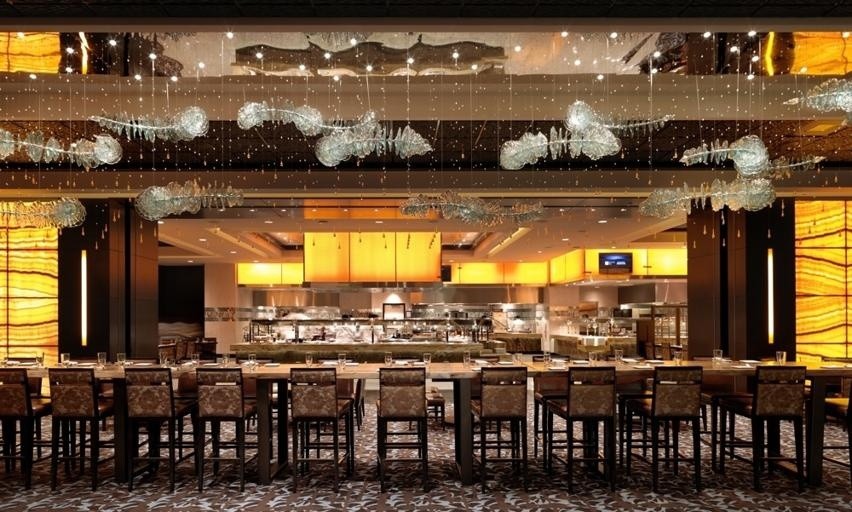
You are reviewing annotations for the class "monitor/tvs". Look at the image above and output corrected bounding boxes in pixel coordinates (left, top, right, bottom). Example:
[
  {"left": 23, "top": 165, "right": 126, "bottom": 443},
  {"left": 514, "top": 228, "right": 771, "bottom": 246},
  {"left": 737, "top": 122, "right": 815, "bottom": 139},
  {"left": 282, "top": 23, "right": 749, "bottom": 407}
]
[{"left": 599, "top": 252, "right": 634, "bottom": 275}]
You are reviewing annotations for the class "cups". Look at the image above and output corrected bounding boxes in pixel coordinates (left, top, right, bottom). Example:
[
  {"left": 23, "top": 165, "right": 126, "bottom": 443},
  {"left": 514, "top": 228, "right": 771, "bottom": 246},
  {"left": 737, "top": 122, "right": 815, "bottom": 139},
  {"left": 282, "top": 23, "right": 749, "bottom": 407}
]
[{"left": 1, "top": 351, "right": 785, "bottom": 373}]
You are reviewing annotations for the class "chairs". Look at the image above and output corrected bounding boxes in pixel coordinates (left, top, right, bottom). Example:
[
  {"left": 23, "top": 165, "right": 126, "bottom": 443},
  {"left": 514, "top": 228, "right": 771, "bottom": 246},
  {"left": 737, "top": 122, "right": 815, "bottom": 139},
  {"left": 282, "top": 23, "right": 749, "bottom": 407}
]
[{"left": 0, "top": 335, "right": 852, "bottom": 492}]
